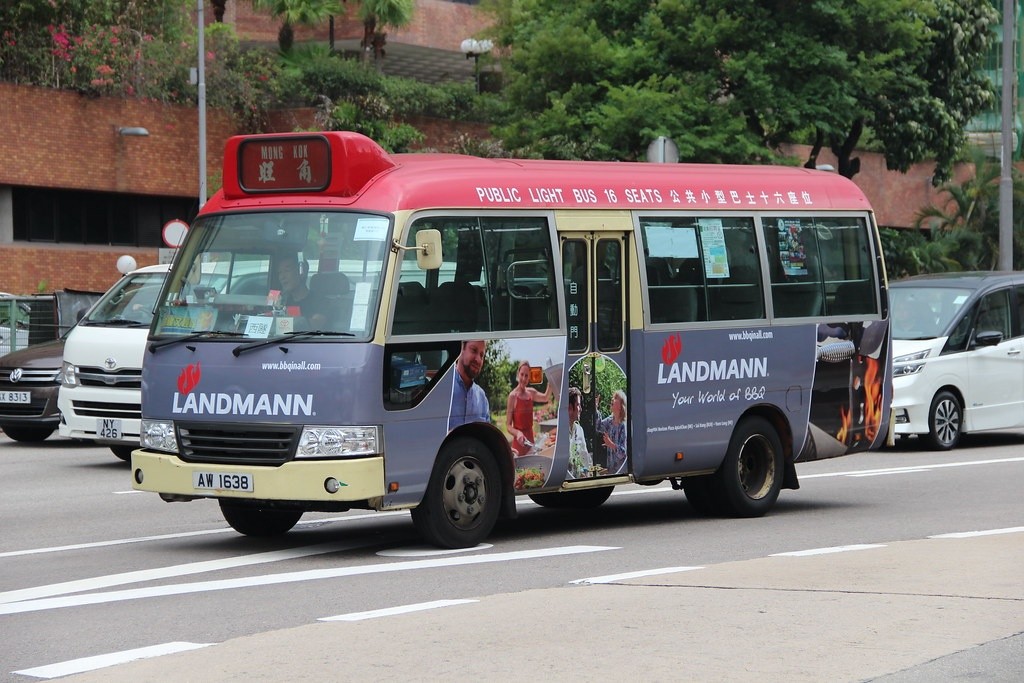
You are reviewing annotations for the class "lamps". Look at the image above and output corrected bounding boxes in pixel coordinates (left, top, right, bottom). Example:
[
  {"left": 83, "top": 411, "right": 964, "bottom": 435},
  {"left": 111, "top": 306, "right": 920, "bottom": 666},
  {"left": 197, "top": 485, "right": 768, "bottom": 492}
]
[{"left": 114, "top": 125, "right": 149, "bottom": 136}]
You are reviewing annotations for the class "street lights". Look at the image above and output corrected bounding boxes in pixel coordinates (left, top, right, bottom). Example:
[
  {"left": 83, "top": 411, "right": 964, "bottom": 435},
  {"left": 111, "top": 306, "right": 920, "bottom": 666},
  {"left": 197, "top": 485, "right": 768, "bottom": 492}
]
[{"left": 460, "top": 38, "right": 494, "bottom": 94}]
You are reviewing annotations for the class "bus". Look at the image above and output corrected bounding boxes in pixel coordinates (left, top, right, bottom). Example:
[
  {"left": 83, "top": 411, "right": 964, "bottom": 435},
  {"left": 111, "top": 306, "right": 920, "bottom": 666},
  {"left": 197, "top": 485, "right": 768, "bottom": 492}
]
[{"left": 131, "top": 130, "right": 893, "bottom": 549}]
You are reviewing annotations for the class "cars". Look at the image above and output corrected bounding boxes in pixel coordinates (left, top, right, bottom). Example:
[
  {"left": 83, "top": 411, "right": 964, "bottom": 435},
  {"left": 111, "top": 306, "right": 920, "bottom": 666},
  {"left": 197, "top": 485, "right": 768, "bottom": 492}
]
[
  {"left": 881, "top": 271, "right": 1024, "bottom": 451},
  {"left": 0, "top": 286, "right": 162, "bottom": 442},
  {"left": 0, "top": 292, "right": 31, "bottom": 357}
]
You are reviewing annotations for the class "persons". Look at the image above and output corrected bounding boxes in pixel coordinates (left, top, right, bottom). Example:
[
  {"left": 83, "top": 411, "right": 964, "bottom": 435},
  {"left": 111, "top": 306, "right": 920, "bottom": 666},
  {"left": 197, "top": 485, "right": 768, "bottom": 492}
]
[
  {"left": 596, "top": 389, "right": 629, "bottom": 472},
  {"left": 568, "top": 388, "right": 595, "bottom": 474},
  {"left": 448, "top": 340, "right": 495, "bottom": 432},
  {"left": 506, "top": 362, "right": 554, "bottom": 457},
  {"left": 266, "top": 254, "right": 328, "bottom": 330}
]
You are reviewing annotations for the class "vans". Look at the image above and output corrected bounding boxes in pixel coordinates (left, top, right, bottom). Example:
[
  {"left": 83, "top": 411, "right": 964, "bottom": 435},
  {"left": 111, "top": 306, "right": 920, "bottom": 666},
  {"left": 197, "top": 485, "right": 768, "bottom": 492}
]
[{"left": 57, "top": 260, "right": 487, "bottom": 460}]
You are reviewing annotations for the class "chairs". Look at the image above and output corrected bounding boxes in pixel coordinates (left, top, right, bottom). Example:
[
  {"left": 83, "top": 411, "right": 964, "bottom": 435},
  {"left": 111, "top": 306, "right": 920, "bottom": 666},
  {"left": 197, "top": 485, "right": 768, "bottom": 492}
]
[
  {"left": 392, "top": 252, "right": 874, "bottom": 335},
  {"left": 310, "top": 272, "right": 356, "bottom": 333}
]
